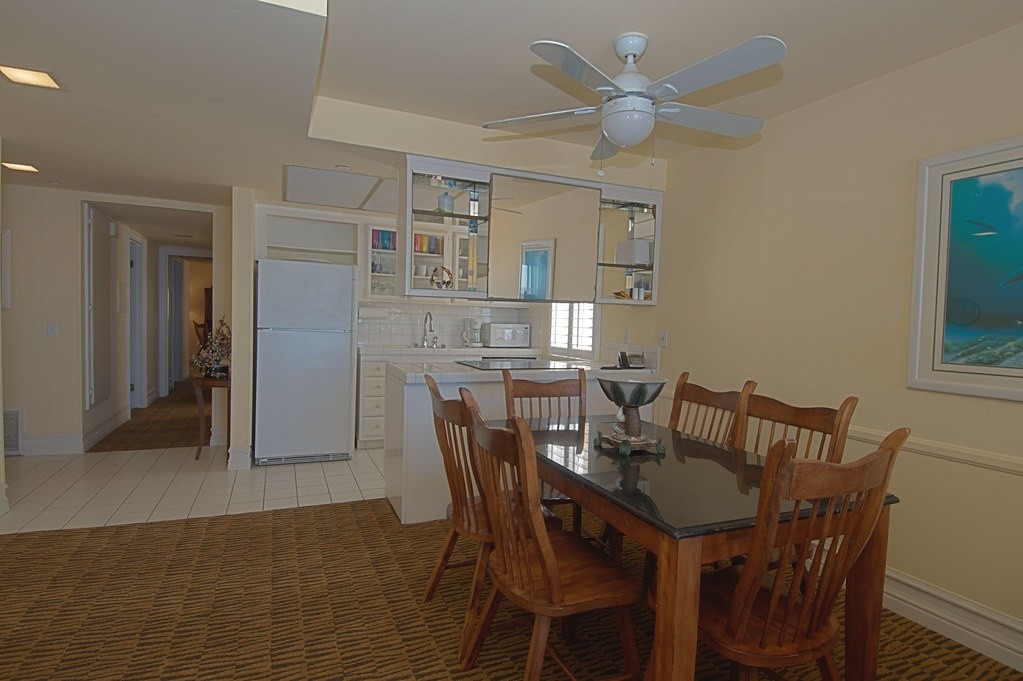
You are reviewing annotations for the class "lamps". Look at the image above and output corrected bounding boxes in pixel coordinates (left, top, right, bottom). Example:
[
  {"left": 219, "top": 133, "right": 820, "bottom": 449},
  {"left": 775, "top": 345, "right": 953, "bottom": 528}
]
[{"left": 600, "top": 97, "right": 657, "bottom": 148}]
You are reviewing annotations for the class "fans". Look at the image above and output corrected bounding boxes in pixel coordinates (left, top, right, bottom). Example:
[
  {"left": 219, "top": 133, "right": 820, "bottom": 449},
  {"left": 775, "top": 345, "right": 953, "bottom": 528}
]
[{"left": 481, "top": 31, "right": 788, "bottom": 160}]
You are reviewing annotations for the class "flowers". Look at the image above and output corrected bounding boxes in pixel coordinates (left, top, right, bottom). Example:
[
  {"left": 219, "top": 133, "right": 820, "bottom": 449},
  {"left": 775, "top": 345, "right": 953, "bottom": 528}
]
[{"left": 190, "top": 316, "right": 232, "bottom": 380}]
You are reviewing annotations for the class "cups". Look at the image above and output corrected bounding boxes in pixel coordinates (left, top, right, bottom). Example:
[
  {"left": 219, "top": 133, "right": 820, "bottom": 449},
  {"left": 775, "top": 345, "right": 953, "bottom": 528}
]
[
  {"left": 414, "top": 234, "right": 444, "bottom": 255},
  {"left": 639, "top": 289, "right": 644, "bottom": 300},
  {"left": 372, "top": 229, "right": 396, "bottom": 251},
  {"left": 372, "top": 254, "right": 396, "bottom": 275},
  {"left": 412, "top": 264, "right": 464, "bottom": 279},
  {"left": 631, "top": 288, "right": 638, "bottom": 300}
]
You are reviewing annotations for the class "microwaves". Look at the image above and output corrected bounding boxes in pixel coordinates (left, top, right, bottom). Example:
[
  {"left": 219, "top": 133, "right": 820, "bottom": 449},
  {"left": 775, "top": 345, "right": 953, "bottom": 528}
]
[{"left": 483, "top": 323, "right": 530, "bottom": 348}]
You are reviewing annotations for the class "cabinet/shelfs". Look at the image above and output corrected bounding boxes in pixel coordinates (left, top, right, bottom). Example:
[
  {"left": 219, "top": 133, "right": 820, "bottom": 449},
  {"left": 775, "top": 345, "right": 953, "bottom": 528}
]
[
  {"left": 360, "top": 157, "right": 489, "bottom": 299},
  {"left": 358, "top": 353, "right": 386, "bottom": 450},
  {"left": 598, "top": 181, "right": 664, "bottom": 307}
]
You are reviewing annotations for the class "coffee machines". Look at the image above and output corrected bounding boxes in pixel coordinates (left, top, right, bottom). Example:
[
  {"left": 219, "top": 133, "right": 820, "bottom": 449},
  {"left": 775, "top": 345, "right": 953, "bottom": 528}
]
[{"left": 463, "top": 317, "right": 483, "bottom": 348}]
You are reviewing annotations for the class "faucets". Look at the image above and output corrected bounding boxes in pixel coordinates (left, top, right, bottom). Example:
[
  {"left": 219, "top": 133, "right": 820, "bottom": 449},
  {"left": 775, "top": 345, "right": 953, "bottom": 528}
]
[{"left": 422, "top": 311, "right": 435, "bottom": 347}]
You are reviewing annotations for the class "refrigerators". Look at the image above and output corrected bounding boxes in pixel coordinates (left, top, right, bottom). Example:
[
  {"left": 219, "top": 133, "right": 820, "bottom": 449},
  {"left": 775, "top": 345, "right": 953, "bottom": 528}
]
[{"left": 253, "top": 256, "right": 358, "bottom": 466}]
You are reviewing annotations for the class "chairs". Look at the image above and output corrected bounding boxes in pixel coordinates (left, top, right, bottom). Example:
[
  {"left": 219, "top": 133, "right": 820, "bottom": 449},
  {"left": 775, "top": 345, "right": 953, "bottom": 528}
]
[{"left": 419, "top": 366, "right": 913, "bottom": 680}]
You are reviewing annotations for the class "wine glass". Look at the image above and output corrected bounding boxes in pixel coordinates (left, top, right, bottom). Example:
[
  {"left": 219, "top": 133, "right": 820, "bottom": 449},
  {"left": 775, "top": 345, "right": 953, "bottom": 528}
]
[{"left": 372, "top": 277, "right": 396, "bottom": 296}]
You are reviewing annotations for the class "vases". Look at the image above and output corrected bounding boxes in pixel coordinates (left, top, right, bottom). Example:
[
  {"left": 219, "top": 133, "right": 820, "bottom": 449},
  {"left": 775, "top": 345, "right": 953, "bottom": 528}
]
[{"left": 206, "top": 365, "right": 228, "bottom": 379}]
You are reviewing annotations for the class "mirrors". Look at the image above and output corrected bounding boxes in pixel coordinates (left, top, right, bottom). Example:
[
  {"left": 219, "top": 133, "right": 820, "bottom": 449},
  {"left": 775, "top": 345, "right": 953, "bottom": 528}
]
[{"left": 490, "top": 172, "right": 601, "bottom": 303}]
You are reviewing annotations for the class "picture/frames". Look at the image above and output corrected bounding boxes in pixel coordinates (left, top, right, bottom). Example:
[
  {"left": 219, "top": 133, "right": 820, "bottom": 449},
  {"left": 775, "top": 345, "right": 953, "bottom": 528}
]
[
  {"left": 518, "top": 238, "right": 556, "bottom": 300},
  {"left": 905, "top": 136, "right": 1023, "bottom": 401}
]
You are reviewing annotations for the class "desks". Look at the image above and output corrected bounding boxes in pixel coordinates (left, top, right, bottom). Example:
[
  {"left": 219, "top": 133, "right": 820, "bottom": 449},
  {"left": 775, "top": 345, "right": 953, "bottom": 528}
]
[
  {"left": 195, "top": 378, "right": 230, "bottom": 460},
  {"left": 482, "top": 416, "right": 899, "bottom": 681}
]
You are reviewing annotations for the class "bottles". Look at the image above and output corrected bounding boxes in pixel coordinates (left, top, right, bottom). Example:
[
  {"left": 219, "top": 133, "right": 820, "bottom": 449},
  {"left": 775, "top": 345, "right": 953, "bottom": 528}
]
[{"left": 437, "top": 192, "right": 455, "bottom": 214}]
